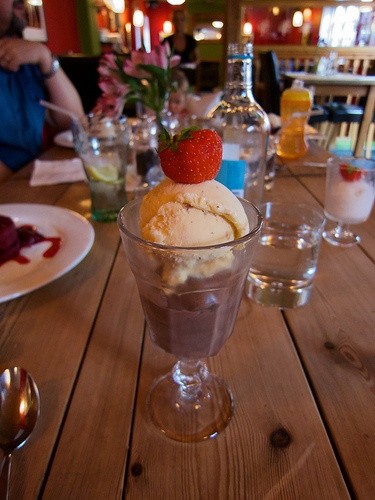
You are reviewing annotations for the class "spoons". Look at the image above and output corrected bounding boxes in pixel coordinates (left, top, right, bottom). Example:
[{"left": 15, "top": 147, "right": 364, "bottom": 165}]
[{"left": 0, "top": 366, "right": 41, "bottom": 500}]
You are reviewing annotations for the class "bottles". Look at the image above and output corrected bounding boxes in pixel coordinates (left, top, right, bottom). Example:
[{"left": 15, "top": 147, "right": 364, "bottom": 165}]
[
  {"left": 275, "top": 80, "right": 313, "bottom": 159},
  {"left": 136, "top": 111, "right": 183, "bottom": 188},
  {"left": 209, "top": 41, "right": 271, "bottom": 207}
]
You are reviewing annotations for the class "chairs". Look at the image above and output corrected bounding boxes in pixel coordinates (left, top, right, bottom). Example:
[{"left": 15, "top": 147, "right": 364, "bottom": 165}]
[{"left": 262, "top": 50, "right": 370, "bottom": 150}]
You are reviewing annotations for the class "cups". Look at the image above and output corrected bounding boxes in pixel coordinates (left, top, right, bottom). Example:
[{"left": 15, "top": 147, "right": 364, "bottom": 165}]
[
  {"left": 244, "top": 201, "right": 326, "bottom": 310},
  {"left": 72, "top": 113, "right": 129, "bottom": 222}
]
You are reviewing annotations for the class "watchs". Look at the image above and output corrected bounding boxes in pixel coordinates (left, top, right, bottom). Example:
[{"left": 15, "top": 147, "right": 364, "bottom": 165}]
[{"left": 45, "top": 55, "right": 60, "bottom": 80}]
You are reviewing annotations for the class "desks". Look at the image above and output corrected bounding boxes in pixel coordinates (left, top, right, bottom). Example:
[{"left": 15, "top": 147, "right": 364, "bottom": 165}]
[
  {"left": 0, "top": 140, "right": 375, "bottom": 500},
  {"left": 284, "top": 72, "right": 375, "bottom": 159}
]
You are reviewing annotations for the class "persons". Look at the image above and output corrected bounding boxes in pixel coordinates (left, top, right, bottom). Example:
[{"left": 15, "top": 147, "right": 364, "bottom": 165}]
[
  {"left": 0, "top": 0, "right": 85, "bottom": 173},
  {"left": 159, "top": 9, "right": 199, "bottom": 94}
]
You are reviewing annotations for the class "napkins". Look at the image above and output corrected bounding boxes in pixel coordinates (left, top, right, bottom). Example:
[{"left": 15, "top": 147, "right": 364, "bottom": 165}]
[{"left": 29, "top": 159, "right": 87, "bottom": 187}]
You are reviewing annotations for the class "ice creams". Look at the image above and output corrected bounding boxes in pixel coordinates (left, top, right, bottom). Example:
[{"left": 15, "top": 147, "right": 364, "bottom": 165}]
[{"left": 135, "top": 179, "right": 249, "bottom": 290}]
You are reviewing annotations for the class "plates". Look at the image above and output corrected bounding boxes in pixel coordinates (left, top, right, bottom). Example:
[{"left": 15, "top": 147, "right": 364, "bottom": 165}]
[
  {"left": 55, "top": 130, "right": 114, "bottom": 149},
  {"left": 0, "top": 202, "right": 95, "bottom": 302}
]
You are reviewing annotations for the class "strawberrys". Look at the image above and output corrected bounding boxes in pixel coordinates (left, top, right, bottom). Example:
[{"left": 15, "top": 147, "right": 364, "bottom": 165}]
[
  {"left": 157, "top": 122, "right": 224, "bottom": 184},
  {"left": 339, "top": 165, "right": 363, "bottom": 181}
]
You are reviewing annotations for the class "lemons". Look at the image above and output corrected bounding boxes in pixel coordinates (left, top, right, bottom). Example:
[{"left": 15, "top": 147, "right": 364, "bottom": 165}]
[{"left": 88, "top": 162, "right": 121, "bottom": 184}]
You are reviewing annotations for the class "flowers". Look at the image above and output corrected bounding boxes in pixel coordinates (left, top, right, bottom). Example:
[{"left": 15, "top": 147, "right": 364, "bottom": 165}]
[{"left": 97, "top": 46, "right": 180, "bottom": 118}]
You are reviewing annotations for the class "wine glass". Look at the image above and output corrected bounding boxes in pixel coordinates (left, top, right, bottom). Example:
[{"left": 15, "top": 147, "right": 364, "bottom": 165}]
[
  {"left": 116, "top": 194, "right": 265, "bottom": 445},
  {"left": 322, "top": 156, "right": 375, "bottom": 248}
]
